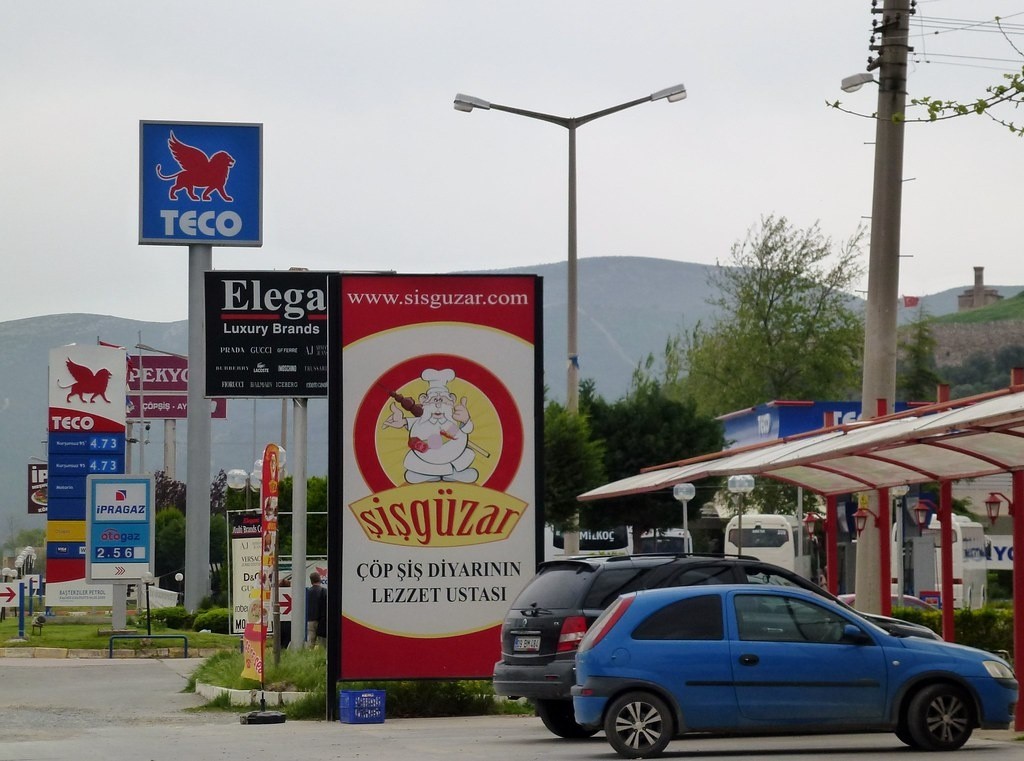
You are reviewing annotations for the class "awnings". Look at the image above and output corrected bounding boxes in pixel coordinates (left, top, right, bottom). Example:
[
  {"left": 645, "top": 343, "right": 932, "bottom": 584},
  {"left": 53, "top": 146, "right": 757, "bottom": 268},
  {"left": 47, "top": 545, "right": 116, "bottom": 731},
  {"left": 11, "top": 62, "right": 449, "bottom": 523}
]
[{"left": 578, "top": 391, "right": 1024, "bottom": 502}]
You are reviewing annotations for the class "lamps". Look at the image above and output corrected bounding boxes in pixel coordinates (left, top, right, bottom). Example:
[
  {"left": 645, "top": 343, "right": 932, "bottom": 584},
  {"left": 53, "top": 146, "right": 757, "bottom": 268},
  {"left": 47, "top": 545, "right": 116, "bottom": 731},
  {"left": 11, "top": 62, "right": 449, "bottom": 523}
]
[
  {"left": 914, "top": 499, "right": 941, "bottom": 534},
  {"left": 852, "top": 507, "right": 879, "bottom": 538},
  {"left": 984, "top": 491, "right": 1012, "bottom": 526},
  {"left": 801, "top": 511, "right": 825, "bottom": 540}
]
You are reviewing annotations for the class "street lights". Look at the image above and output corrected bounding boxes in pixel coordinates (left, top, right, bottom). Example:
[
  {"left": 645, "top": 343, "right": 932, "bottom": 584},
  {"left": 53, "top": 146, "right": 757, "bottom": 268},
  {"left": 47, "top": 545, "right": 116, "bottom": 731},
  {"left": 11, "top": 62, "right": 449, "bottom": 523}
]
[
  {"left": 455, "top": 82, "right": 690, "bottom": 556},
  {"left": 673, "top": 483, "right": 696, "bottom": 557},
  {"left": 226, "top": 468, "right": 263, "bottom": 510},
  {"left": 838, "top": 73, "right": 908, "bottom": 619},
  {"left": 727, "top": 474, "right": 755, "bottom": 559}
]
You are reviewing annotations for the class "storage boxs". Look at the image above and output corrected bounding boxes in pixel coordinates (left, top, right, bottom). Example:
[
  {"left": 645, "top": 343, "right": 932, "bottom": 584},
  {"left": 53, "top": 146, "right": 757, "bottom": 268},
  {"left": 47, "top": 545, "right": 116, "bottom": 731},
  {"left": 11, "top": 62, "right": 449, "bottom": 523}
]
[{"left": 338, "top": 690, "right": 387, "bottom": 723}]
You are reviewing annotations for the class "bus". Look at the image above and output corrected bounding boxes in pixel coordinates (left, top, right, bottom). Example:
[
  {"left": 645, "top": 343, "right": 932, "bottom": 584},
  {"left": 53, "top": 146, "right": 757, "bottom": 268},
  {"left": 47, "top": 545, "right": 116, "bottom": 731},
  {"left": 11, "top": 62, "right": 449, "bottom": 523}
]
[
  {"left": 634, "top": 527, "right": 694, "bottom": 555},
  {"left": 544, "top": 519, "right": 634, "bottom": 561},
  {"left": 723, "top": 513, "right": 830, "bottom": 610}
]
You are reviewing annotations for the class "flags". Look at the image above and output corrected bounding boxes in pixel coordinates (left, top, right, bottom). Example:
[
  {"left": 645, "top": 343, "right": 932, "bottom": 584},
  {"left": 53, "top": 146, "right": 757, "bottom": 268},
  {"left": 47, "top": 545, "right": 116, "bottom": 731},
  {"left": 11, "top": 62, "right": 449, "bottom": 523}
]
[{"left": 903, "top": 297, "right": 919, "bottom": 307}]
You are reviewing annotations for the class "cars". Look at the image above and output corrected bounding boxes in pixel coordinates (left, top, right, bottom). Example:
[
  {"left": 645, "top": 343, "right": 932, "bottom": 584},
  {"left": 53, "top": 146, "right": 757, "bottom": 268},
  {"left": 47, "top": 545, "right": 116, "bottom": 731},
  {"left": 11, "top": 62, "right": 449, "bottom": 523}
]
[
  {"left": 837, "top": 592, "right": 938, "bottom": 614},
  {"left": 569, "top": 583, "right": 1020, "bottom": 759}
]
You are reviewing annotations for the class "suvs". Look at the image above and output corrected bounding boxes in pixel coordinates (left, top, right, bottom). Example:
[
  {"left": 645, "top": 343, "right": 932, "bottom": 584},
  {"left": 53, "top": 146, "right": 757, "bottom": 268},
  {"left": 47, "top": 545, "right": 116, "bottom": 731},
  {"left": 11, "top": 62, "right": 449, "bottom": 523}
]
[{"left": 491, "top": 550, "right": 947, "bottom": 740}]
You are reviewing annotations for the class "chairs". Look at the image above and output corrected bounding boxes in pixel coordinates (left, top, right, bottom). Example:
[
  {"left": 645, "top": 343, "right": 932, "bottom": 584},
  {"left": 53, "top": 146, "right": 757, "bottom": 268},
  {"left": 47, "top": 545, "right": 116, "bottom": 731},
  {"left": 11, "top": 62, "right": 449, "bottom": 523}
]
[{"left": 756, "top": 604, "right": 801, "bottom": 632}]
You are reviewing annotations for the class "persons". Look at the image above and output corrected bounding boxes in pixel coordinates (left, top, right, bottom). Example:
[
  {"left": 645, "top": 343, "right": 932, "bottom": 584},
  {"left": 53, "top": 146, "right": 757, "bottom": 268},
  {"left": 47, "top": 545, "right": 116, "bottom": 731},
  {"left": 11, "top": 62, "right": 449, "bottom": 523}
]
[
  {"left": 46, "top": 606, "right": 52, "bottom": 616},
  {"left": 279, "top": 573, "right": 327, "bottom": 650}
]
